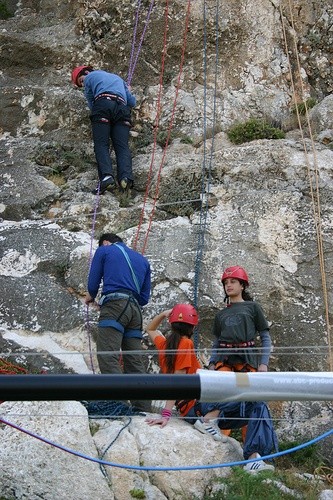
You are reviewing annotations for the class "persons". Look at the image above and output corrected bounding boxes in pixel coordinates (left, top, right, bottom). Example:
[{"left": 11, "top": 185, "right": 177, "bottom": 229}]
[
  {"left": 208, "top": 265, "right": 272, "bottom": 446},
  {"left": 85, "top": 233, "right": 152, "bottom": 413},
  {"left": 145, "top": 304, "right": 275, "bottom": 476},
  {"left": 72, "top": 66, "right": 137, "bottom": 194}
]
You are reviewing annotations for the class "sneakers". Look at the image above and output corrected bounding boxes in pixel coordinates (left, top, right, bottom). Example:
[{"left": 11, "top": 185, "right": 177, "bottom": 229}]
[
  {"left": 194, "top": 417, "right": 222, "bottom": 441},
  {"left": 242, "top": 453, "right": 274, "bottom": 472}
]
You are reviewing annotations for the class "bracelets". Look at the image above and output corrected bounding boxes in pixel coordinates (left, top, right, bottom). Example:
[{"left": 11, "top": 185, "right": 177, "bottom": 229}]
[{"left": 162, "top": 409, "right": 172, "bottom": 418}]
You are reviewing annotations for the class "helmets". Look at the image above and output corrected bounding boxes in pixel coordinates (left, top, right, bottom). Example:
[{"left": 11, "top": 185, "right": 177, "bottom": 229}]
[
  {"left": 169, "top": 304, "right": 199, "bottom": 326},
  {"left": 73, "top": 65, "right": 90, "bottom": 88},
  {"left": 221, "top": 267, "right": 250, "bottom": 287}
]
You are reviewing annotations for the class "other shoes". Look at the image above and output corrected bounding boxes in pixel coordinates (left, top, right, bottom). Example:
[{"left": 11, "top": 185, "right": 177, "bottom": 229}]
[
  {"left": 93, "top": 177, "right": 114, "bottom": 195},
  {"left": 119, "top": 176, "right": 133, "bottom": 191}
]
